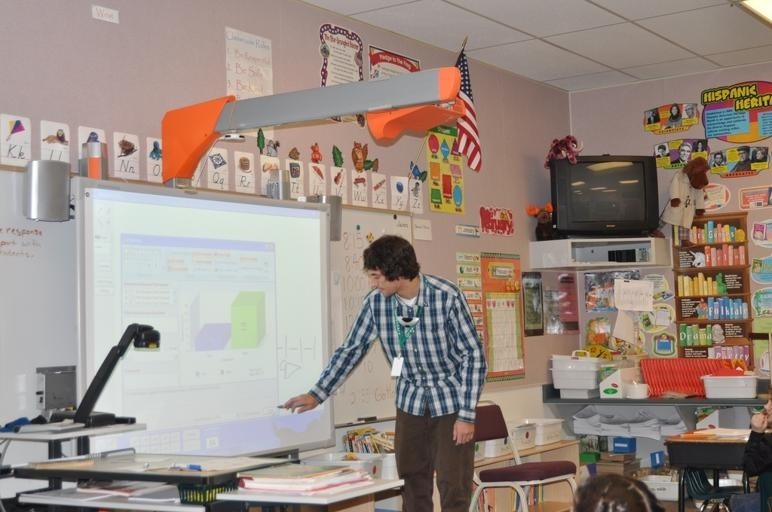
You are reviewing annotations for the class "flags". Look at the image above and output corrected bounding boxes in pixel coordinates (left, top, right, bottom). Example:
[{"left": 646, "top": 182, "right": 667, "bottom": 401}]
[{"left": 446, "top": 52, "right": 482, "bottom": 174}]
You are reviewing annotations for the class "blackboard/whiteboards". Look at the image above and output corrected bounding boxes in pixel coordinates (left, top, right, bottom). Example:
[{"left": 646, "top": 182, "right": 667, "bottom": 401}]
[
  {"left": 0, "top": 166, "right": 413, "bottom": 500},
  {"left": 293, "top": 418, "right": 400, "bottom": 463}
]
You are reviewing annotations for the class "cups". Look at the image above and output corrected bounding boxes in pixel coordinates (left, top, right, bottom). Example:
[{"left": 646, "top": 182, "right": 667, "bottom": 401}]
[{"left": 625, "top": 384, "right": 652, "bottom": 399}]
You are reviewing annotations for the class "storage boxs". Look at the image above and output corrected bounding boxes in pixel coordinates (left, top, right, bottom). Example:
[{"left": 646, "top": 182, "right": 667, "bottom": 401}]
[
  {"left": 507, "top": 417, "right": 566, "bottom": 449},
  {"left": 547, "top": 348, "right": 601, "bottom": 391},
  {"left": 503, "top": 420, "right": 537, "bottom": 452},
  {"left": 297, "top": 450, "right": 383, "bottom": 483},
  {"left": 379, "top": 453, "right": 400, "bottom": 482},
  {"left": 700, "top": 373, "right": 760, "bottom": 399}
]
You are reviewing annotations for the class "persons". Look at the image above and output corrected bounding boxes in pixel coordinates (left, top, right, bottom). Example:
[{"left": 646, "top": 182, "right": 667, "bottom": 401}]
[
  {"left": 730, "top": 398, "right": 772, "bottom": 512},
  {"left": 646, "top": 104, "right": 768, "bottom": 173},
  {"left": 284, "top": 234, "right": 487, "bottom": 512}
]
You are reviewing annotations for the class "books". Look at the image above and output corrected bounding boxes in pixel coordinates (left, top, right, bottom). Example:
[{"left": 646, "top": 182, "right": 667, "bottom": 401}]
[
  {"left": 212, "top": 463, "right": 377, "bottom": 495},
  {"left": 19, "top": 482, "right": 180, "bottom": 502},
  {"left": 340, "top": 426, "right": 395, "bottom": 454},
  {"left": 29, "top": 459, "right": 94, "bottom": 470}
]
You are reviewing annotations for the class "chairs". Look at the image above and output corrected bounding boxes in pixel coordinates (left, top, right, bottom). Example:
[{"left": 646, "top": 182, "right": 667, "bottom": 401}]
[{"left": 464, "top": 400, "right": 581, "bottom": 511}]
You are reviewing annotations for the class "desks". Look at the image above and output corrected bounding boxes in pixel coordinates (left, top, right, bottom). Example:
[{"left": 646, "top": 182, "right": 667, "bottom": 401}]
[
  {"left": 662, "top": 426, "right": 752, "bottom": 512},
  {"left": 471, "top": 439, "right": 584, "bottom": 473},
  {"left": 14, "top": 453, "right": 294, "bottom": 487}
]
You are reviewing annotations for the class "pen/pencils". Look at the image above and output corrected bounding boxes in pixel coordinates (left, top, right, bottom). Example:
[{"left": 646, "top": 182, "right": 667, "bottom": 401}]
[
  {"left": 347, "top": 421, "right": 365, "bottom": 424},
  {"left": 277, "top": 405, "right": 303, "bottom": 409}
]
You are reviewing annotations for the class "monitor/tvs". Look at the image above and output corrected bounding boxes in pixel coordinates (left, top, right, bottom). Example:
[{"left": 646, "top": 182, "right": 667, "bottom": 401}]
[{"left": 549, "top": 154, "right": 660, "bottom": 239}]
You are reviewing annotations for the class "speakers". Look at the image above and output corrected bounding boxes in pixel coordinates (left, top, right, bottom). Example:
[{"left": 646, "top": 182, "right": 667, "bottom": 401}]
[
  {"left": 22, "top": 159, "right": 72, "bottom": 223},
  {"left": 303, "top": 194, "right": 343, "bottom": 240}
]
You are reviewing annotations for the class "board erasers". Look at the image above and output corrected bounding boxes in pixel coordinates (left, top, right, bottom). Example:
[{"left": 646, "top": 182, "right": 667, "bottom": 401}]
[{"left": 358, "top": 417, "right": 376, "bottom": 422}]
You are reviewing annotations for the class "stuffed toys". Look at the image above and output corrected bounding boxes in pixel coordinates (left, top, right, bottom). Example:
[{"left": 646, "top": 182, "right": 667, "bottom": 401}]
[
  {"left": 526, "top": 202, "right": 555, "bottom": 240},
  {"left": 543, "top": 134, "right": 585, "bottom": 170},
  {"left": 658, "top": 156, "right": 711, "bottom": 230}
]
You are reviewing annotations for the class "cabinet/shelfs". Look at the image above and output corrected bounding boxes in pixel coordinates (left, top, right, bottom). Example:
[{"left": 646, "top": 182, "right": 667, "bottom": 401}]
[
  {"left": 527, "top": 236, "right": 674, "bottom": 270},
  {"left": 670, "top": 209, "right": 756, "bottom": 372}
]
